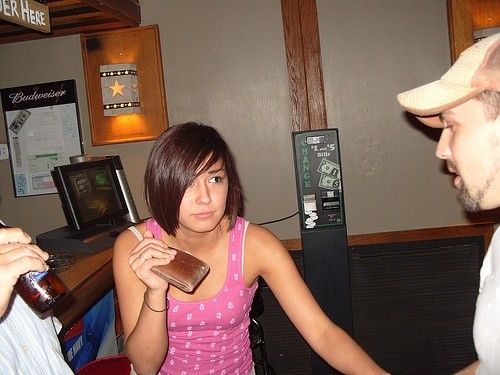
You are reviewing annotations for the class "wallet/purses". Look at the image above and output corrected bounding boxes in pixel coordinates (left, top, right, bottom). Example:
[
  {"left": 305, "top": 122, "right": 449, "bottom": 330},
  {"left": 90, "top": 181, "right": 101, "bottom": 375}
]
[{"left": 152, "top": 245, "right": 212, "bottom": 294}]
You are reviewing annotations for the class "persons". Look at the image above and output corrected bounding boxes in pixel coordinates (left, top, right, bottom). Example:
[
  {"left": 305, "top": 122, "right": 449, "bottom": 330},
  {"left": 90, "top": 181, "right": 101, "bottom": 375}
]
[
  {"left": 112, "top": 122, "right": 391, "bottom": 374},
  {"left": 397, "top": 33, "right": 500, "bottom": 375},
  {"left": 0, "top": 218, "right": 75, "bottom": 375}
]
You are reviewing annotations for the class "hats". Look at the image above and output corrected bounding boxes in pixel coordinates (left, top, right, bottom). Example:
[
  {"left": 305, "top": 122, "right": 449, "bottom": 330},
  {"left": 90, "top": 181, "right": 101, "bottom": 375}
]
[{"left": 397, "top": 33, "right": 500, "bottom": 128}]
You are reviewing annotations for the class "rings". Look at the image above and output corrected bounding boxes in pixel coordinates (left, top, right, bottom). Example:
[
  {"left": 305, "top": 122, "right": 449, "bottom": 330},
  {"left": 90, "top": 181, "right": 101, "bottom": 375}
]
[{"left": 140, "top": 256, "right": 146, "bottom": 260}]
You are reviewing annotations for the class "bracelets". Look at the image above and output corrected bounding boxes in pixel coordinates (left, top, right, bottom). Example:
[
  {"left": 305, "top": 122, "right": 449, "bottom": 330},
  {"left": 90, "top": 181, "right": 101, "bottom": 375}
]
[{"left": 144, "top": 291, "right": 167, "bottom": 312}]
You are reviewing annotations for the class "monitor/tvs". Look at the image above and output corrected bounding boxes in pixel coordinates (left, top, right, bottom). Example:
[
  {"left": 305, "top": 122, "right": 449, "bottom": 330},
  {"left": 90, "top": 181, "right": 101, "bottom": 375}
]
[{"left": 54, "top": 159, "right": 129, "bottom": 232}]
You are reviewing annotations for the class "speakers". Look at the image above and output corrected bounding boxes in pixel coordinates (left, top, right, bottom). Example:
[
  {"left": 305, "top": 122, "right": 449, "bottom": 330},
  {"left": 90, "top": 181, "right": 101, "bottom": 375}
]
[
  {"left": 299, "top": 227, "right": 353, "bottom": 375},
  {"left": 292, "top": 128, "right": 346, "bottom": 228}
]
